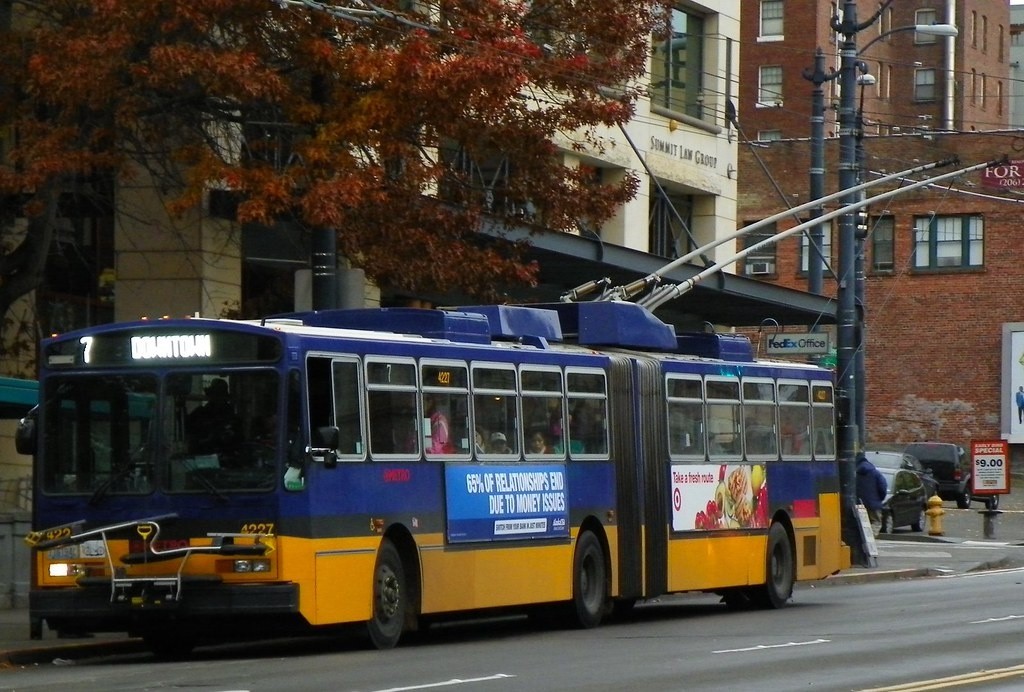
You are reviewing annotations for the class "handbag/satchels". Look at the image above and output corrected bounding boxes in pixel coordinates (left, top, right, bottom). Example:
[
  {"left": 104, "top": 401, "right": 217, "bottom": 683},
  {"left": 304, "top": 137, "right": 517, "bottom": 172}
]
[{"left": 442, "top": 434, "right": 457, "bottom": 454}]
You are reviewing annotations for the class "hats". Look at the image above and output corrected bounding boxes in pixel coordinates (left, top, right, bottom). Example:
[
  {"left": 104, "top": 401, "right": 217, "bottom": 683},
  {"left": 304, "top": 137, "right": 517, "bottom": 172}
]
[
  {"left": 491, "top": 432, "right": 507, "bottom": 443},
  {"left": 203, "top": 378, "right": 231, "bottom": 401}
]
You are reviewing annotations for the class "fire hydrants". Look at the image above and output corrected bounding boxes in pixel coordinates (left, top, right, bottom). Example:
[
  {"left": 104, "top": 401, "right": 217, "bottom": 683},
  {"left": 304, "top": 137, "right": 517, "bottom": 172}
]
[{"left": 926, "top": 490, "right": 946, "bottom": 539}]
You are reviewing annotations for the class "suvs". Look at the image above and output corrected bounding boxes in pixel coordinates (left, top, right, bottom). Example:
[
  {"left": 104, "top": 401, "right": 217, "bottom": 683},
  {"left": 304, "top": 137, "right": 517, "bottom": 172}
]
[{"left": 903, "top": 442, "right": 1001, "bottom": 509}]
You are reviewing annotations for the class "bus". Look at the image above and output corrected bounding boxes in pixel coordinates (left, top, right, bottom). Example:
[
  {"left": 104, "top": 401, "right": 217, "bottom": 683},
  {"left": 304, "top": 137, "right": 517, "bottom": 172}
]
[{"left": 15, "top": 301, "right": 854, "bottom": 652}]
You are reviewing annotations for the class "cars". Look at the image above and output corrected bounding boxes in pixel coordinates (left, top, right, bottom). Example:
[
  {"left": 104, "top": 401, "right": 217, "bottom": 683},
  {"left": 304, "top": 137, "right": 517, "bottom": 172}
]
[
  {"left": 863, "top": 450, "right": 940, "bottom": 510},
  {"left": 872, "top": 467, "right": 927, "bottom": 535}
]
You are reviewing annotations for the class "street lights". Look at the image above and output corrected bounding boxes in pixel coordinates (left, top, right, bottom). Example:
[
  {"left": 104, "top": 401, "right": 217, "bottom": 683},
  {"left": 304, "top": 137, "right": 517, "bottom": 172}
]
[{"left": 804, "top": 22, "right": 959, "bottom": 365}]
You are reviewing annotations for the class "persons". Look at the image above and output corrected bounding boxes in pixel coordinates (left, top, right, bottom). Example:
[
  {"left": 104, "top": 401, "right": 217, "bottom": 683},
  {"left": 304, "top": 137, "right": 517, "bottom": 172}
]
[
  {"left": 856, "top": 452, "right": 888, "bottom": 539},
  {"left": 1015, "top": 386, "right": 1024, "bottom": 424},
  {"left": 182, "top": 378, "right": 246, "bottom": 468},
  {"left": 371, "top": 376, "right": 606, "bottom": 456},
  {"left": 117, "top": 414, "right": 187, "bottom": 493}
]
